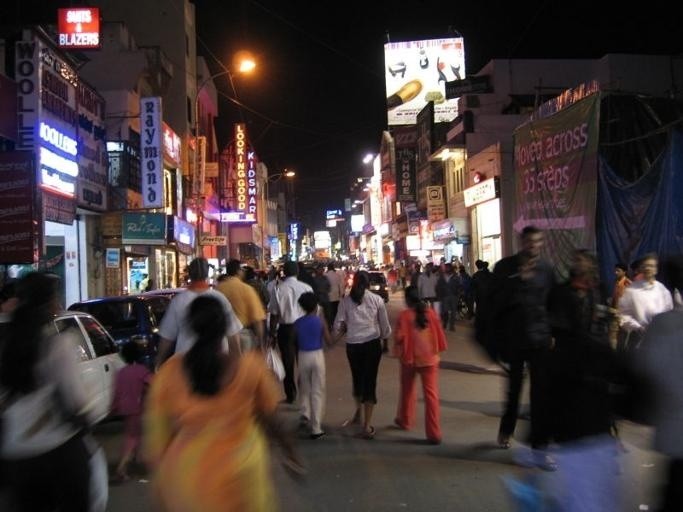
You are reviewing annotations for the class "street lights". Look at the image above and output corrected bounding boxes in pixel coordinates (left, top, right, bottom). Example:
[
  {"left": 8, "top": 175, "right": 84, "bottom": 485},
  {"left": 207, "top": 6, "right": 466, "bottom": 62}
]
[
  {"left": 260, "top": 171, "right": 294, "bottom": 268},
  {"left": 191, "top": 59, "right": 255, "bottom": 263}
]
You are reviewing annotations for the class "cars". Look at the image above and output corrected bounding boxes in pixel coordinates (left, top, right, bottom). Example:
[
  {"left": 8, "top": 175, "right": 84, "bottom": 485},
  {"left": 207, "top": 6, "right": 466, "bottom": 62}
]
[
  {"left": 50, "top": 311, "right": 130, "bottom": 434},
  {"left": 250, "top": 258, "right": 388, "bottom": 301},
  {"left": 68, "top": 288, "right": 188, "bottom": 429}
]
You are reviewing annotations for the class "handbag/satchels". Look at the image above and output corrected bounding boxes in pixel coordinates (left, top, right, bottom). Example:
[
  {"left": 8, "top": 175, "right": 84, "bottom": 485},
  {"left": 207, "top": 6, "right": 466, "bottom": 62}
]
[
  {"left": 0, "top": 381, "right": 89, "bottom": 460},
  {"left": 265, "top": 345, "right": 286, "bottom": 383}
]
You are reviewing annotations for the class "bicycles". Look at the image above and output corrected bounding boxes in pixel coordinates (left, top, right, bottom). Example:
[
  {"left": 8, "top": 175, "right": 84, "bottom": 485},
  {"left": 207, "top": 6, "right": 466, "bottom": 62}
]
[{"left": 457, "top": 295, "right": 468, "bottom": 318}]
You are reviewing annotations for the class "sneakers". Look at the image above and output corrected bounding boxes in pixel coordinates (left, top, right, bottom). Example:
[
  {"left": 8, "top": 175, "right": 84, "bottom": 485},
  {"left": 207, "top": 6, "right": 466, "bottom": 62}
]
[
  {"left": 425, "top": 434, "right": 444, "bottom": 446},
  {"left": 298, "top": 417, "right": 307, "bottom": 431},
  {"left": 533, "top": 452, "right": 559, "bottom": 472},
  {"left": 362, "top": 425, "right": 376, "bottom": 441},
  {"left": 393, "top": 417, "right": 414, "bottom": 431},
  {"left": 497, "top": 433, "right": 513, "bottom": 449},
  {"left": 311, "top": 432, "right": 329, "bottom": 441}
]
